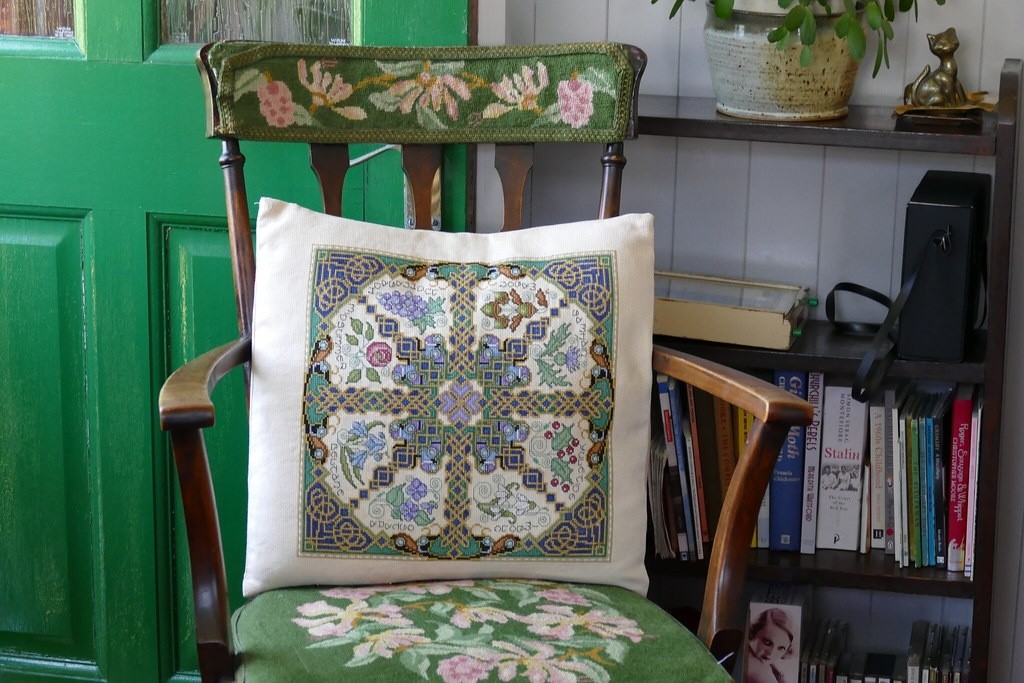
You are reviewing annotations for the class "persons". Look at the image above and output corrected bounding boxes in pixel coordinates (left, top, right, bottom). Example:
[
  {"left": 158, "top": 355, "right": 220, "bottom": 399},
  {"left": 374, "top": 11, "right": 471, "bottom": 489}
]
[
  {"left": 745, "top": 607, "right": 795, "bottom": 683},
  {"left": 836, "top": 467, "right": 851, "bottom": 490},
  {"left": 822, "top": 466, "right": 839, "bottom": 490}
]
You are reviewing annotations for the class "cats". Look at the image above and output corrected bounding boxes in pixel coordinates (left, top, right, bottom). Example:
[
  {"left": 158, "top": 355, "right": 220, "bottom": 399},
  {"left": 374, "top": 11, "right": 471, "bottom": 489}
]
[{"left": 910, "top": 28, "right": 967, "bottom": 108}]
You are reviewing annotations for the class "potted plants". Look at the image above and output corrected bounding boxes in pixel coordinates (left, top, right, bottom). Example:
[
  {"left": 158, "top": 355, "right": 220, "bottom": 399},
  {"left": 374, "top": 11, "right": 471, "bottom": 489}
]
[{"left": 651, "top": 0, "right": 946, "bottom": 122}]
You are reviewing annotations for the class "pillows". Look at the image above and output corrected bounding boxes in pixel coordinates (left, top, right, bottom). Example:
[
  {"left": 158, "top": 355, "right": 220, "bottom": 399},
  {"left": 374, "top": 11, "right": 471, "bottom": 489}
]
[{"left": 242, "top": 196, "right": 654, "bottom": 601}]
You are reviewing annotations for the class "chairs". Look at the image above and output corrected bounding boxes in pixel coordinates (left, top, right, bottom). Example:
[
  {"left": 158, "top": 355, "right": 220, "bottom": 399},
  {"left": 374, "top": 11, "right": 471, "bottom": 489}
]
[{"left": 156, "top": 39, "right": 816, "bottom": 683}]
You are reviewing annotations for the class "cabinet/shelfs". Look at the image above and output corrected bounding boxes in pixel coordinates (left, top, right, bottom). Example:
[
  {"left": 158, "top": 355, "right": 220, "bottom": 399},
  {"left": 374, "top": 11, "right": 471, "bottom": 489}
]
[{"left": 637, "top": 56, "right": 1022, "bottom": 683}]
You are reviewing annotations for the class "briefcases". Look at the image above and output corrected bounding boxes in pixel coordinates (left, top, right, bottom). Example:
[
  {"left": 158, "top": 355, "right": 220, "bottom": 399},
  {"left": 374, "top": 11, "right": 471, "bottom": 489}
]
[{"left": 826, "top": 169, "right": 992, "bottom": 406}]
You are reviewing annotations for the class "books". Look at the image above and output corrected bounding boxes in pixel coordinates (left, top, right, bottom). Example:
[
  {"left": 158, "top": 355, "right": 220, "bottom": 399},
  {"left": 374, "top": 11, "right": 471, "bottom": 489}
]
[
  {"left": 732, "top": 583, "right": 971, "bottom": 683},
  {"left": 648, "top": 361, "right": 984, "bottom": 580}
]
